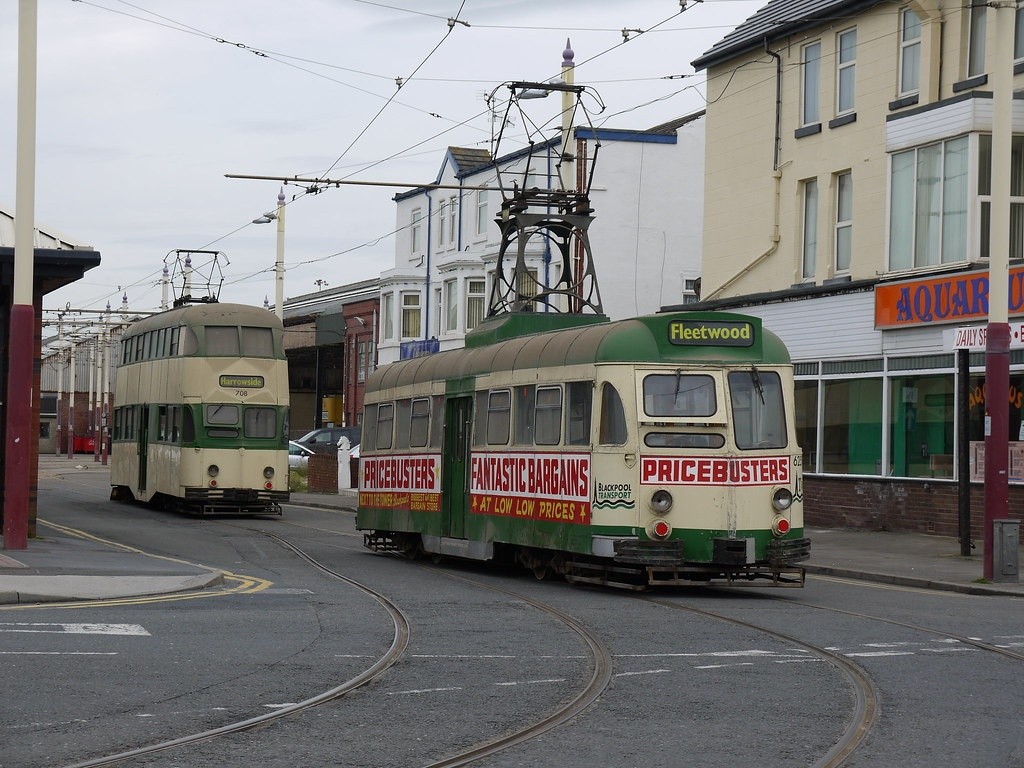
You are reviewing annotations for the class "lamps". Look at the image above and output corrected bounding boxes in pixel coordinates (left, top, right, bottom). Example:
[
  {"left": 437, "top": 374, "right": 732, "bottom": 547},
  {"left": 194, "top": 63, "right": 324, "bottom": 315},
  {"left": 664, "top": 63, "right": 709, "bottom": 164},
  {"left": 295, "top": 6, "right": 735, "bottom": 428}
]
[{"left": 354, "top": 317, "right": 366, "bottom": 325}]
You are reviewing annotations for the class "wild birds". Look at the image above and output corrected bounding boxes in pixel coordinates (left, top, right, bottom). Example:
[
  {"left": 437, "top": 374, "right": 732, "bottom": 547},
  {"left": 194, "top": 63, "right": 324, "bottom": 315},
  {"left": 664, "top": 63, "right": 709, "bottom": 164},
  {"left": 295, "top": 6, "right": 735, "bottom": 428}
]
[
  {"left": 622, "top": 27, "right": 630, "bottom": 42},
  {"left": 263, "top": 213, "right": 278, "bottom": 219},
  {"left": 447, "top": 16, "right": 455, "bottom": 32},
  {"left": 252, "top": 218, "right": 271, "bottom": 224},
  {"left": 679, "top": 0, "right": 688, "bottom": 12},
  {"left": 396, "top": 76, "right": 402, "bottom": 89}
]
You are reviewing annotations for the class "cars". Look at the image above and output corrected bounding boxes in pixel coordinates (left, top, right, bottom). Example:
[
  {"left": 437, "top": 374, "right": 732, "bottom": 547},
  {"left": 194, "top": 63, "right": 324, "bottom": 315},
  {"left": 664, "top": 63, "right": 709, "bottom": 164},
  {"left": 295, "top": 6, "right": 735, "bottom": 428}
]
[{"left": 288, "top": 424, "right": 361, "bottom": 472}]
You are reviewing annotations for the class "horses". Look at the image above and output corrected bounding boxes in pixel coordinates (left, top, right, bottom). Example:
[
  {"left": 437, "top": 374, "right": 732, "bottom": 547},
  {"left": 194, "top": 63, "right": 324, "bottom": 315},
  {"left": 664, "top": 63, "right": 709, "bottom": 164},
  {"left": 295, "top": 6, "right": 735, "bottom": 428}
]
[{"left": 336, "top": 436, "right": 351, "bottom": 449}]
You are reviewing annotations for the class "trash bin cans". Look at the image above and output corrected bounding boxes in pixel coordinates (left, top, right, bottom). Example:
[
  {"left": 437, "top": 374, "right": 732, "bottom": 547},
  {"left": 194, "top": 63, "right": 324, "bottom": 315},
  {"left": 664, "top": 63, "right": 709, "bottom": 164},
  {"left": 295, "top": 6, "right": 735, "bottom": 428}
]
[{"left": 74, "top": 433, "right": 96, "bottom": 453}]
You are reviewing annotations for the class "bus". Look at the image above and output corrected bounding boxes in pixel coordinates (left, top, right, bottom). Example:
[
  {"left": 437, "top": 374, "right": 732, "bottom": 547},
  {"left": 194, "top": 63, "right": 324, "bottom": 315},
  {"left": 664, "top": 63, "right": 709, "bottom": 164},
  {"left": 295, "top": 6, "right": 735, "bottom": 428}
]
[
  {"left": 108, "top": 248, "right": 294, "bottom": 519},
  {"left": 352, "top": 81, "right": 814, "bottom": 598}
]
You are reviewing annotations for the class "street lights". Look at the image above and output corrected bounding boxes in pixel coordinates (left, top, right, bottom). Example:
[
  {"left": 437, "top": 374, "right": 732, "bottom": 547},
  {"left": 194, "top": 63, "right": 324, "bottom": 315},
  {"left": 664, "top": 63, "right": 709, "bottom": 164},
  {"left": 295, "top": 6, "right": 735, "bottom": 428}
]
[{"left": 253, "top": 186, "right": 286, "bottom": 327}]
